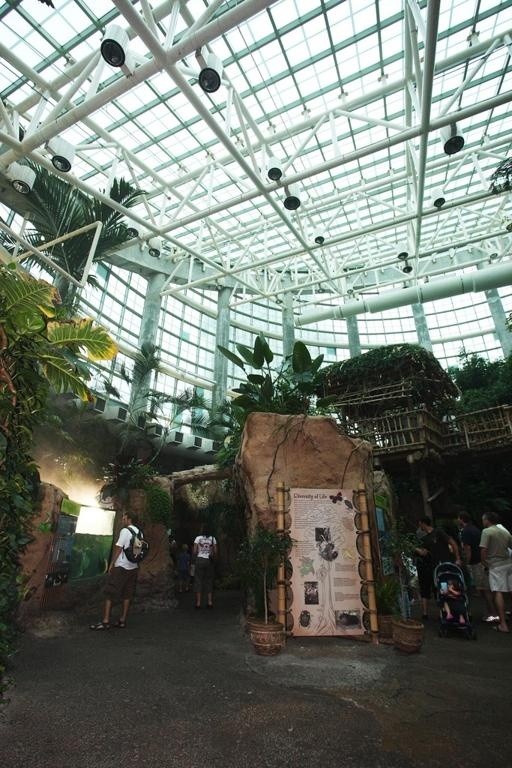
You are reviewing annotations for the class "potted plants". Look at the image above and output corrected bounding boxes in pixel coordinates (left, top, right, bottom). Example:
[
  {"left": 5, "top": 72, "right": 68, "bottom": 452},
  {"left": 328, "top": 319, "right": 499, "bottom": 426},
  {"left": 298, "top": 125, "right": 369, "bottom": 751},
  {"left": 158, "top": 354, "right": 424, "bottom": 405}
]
[
  {"left": 237, "top": 527, "right": 284, "bottom": 656},
  {"left": 380, "top": 519, "right": 425, "bottom": 654}
]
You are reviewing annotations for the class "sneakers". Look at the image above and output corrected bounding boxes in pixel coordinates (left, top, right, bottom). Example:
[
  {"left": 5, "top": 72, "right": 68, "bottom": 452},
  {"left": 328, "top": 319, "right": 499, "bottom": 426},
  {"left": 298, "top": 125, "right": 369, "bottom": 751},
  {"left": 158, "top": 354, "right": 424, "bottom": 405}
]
[
  {"left": 480, "top": 613, "right": 501, "bottom": 622},
  {"left": 445, "top": 612, "right": 453, "bottom": 621},
  {"left": 459, "top": 617, "right": 466, "bottom": 625}
]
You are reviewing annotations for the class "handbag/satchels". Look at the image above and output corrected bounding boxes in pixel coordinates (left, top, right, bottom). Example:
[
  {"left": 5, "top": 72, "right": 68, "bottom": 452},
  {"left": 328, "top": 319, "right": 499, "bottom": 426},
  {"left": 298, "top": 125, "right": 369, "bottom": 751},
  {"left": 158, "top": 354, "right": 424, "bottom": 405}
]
[
  {"left": 209, "top": 545, "right": 214, "bottom": 559},
  {"left": 189, "top": 564, "right": 196, "bottom": 577}
]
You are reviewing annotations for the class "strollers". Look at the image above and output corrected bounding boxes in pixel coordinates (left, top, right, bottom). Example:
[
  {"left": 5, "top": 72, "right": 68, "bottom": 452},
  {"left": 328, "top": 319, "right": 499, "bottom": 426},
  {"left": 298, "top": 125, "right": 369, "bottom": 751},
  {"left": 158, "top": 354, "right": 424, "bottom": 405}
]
[{"left": 432, "top": 558, "right": 478, "bottom": 642}]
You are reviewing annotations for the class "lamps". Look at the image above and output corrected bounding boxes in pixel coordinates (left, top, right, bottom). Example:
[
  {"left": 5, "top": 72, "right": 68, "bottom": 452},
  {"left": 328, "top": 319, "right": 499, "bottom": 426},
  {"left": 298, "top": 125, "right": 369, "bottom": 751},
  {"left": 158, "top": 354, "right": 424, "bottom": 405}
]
[
  {"left": 146, "top": 240, "right": 162, "bottom": 258},
  {"left": 44, "top": 135, "right": 77, "bottom": 172},
  {"left": 8, "top": 164, "right": 38, "bottom": 194},
  {"left": 100, "top": 25, "right": 136, "bottom": 77},
  {"left": 486, "top": 247, "right": 498, "bottom": 258},
  {"left": 261, "top": 157, "right": 283, "bottom": 182},
  {"left": 126, "top": 219, "right": 143, "bottom": 241},
  {"left": 504, "top": 217, "right": 512, "bottom": 230},
  {"left": 401, "top": 260, "right": 412, "bottom": 273},
  {"left": 431, "top": 187, "right": 445, "bottom": 207},
  {"left": 314, "top": 232, "right": 324, "bottom": 245},
  {"left": 440, "top": 125, "right": 465, "bottom": 156},
  {"left": 198, "top": 53, "right": 224, "bottom": 94},
  {"left": 395, "top": 243, "right": 409, "bottom": 261},
  {"left": 282, "top": 190, "right": 300, "bottom": 211}
]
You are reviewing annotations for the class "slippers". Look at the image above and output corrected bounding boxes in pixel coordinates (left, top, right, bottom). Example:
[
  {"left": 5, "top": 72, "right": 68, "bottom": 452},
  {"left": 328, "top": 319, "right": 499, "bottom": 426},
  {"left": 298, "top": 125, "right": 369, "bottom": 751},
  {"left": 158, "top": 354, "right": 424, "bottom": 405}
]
[{"left": 195, "top": 605, "right": 214, "bottom": 609}]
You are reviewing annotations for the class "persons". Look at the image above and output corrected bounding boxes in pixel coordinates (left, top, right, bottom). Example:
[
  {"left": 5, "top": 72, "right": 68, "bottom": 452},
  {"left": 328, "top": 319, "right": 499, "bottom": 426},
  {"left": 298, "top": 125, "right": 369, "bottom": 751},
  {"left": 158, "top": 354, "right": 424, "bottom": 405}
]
[
  {"left": 412, "top": 512, "right": 512, "bottom": 634},
  {"left": 192, "top": 520, "right": 217, "bottom": 608},
  {"left": 89, "top": 510, "right": 143, "bottom": 631},
  {"left": 177, "top": 543, "right": 191, "bottom": 592}
]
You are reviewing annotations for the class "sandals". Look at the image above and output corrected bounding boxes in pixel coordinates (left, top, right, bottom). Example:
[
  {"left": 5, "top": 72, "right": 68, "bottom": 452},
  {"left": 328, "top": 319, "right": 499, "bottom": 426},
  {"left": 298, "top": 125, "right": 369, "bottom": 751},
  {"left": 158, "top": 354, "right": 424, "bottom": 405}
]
[
  {"left": 88, "top": 621, "right": 111, "bottom": 631},
  {"left": 113, "top": 620, "right": 126, "bottom": 628},
  {"left": 492, "top": 624, "right": 510, "bottom": 633}
]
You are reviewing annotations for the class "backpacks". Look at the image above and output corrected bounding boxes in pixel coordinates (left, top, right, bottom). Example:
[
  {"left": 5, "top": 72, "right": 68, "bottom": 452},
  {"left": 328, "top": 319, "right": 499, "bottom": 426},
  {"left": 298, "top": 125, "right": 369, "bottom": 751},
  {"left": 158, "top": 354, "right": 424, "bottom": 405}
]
[{"left": 125, "top": 534, "right": 149, "bottom": 563}]
